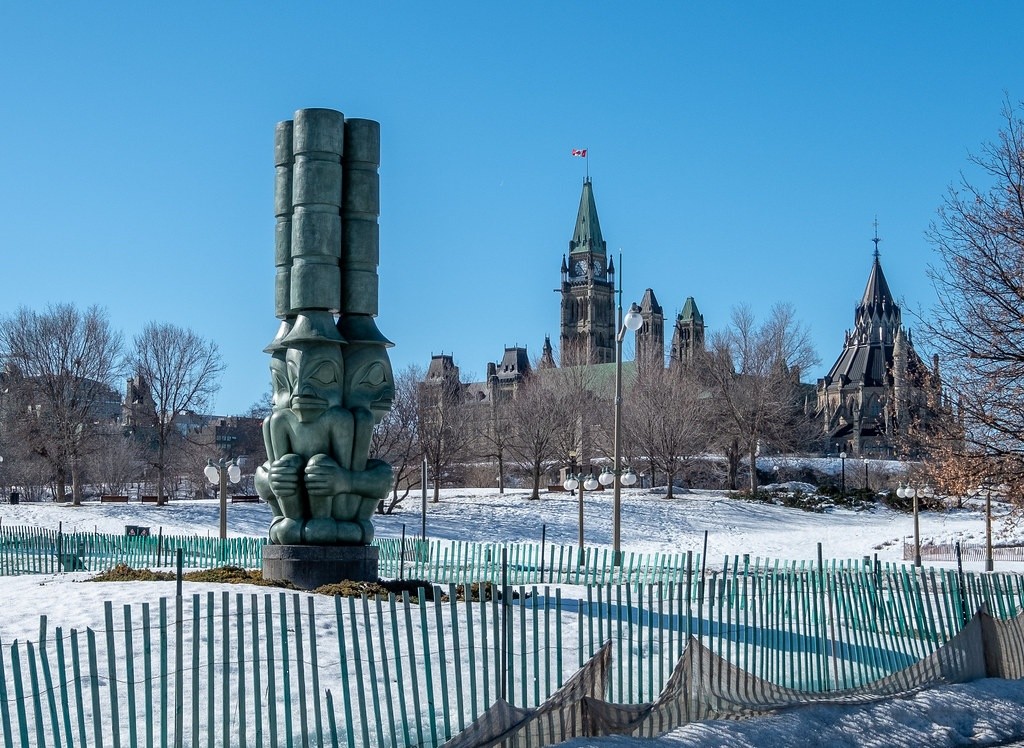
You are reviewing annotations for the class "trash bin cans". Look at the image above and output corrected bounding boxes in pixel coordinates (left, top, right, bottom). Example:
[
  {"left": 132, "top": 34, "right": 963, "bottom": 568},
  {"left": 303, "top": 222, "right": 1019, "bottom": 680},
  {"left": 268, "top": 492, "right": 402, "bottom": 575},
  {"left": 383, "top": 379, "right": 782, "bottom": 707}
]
[
  {"left": 124, "top": 525, "right": 138, "bottom": 536},
  {"left": 136, "top": 527, "right": 151, "bottom": 537}
]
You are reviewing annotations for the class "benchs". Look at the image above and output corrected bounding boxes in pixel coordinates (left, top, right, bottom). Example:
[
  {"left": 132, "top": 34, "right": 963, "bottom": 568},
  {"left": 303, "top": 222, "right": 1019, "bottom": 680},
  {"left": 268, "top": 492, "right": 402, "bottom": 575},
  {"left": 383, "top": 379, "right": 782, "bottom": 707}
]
[
  {"left": 101, "top": 495, "right": 260, "bottom": 503},
  {"left": 547, "top": 485, "right": 570, "bottom": 492}
]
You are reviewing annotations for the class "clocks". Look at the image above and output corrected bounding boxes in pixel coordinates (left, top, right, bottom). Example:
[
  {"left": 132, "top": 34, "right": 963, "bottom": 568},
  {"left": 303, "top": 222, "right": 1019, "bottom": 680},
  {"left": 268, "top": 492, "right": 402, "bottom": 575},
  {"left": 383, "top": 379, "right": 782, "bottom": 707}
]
[
  {"left": 594, "top": 260, "right": 603, "bottom": 276},
  {"left": 575, "top": 260, "right": 588, "bottom": 276}
]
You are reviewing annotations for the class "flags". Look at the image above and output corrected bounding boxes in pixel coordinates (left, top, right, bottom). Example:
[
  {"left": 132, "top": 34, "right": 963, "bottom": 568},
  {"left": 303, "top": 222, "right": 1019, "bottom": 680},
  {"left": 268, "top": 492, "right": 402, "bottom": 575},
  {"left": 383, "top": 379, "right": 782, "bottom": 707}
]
[{"left": 572, "top": 148, "right": 587, "bottom": 158}]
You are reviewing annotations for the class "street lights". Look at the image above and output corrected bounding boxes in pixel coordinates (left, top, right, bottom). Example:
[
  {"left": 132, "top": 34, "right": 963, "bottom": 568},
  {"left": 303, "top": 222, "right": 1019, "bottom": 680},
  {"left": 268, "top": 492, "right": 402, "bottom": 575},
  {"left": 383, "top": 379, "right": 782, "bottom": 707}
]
[
  {"left": 966, "top": 478, "right": 1010, "bottom": 573},
  {"left": 600, "top": 466, "right": 637, "bottom": 563},
  {"left": 562, "top": 471, "right": 598, "bottom": 563},
  {"left": 896, "top": 479, "right": 934, "bottom": 567},
  {"left": 204, "top": 456, "right": 241, "bottom": 538},
  {"left": 612, "top": 252, "right": 645, "bottom": 562},
  {"left": 839, "top": 451, "right": 847, "bottom": 495},
  {"left": 864, "top": 457, "right": 870, "bottom": 492}
]
